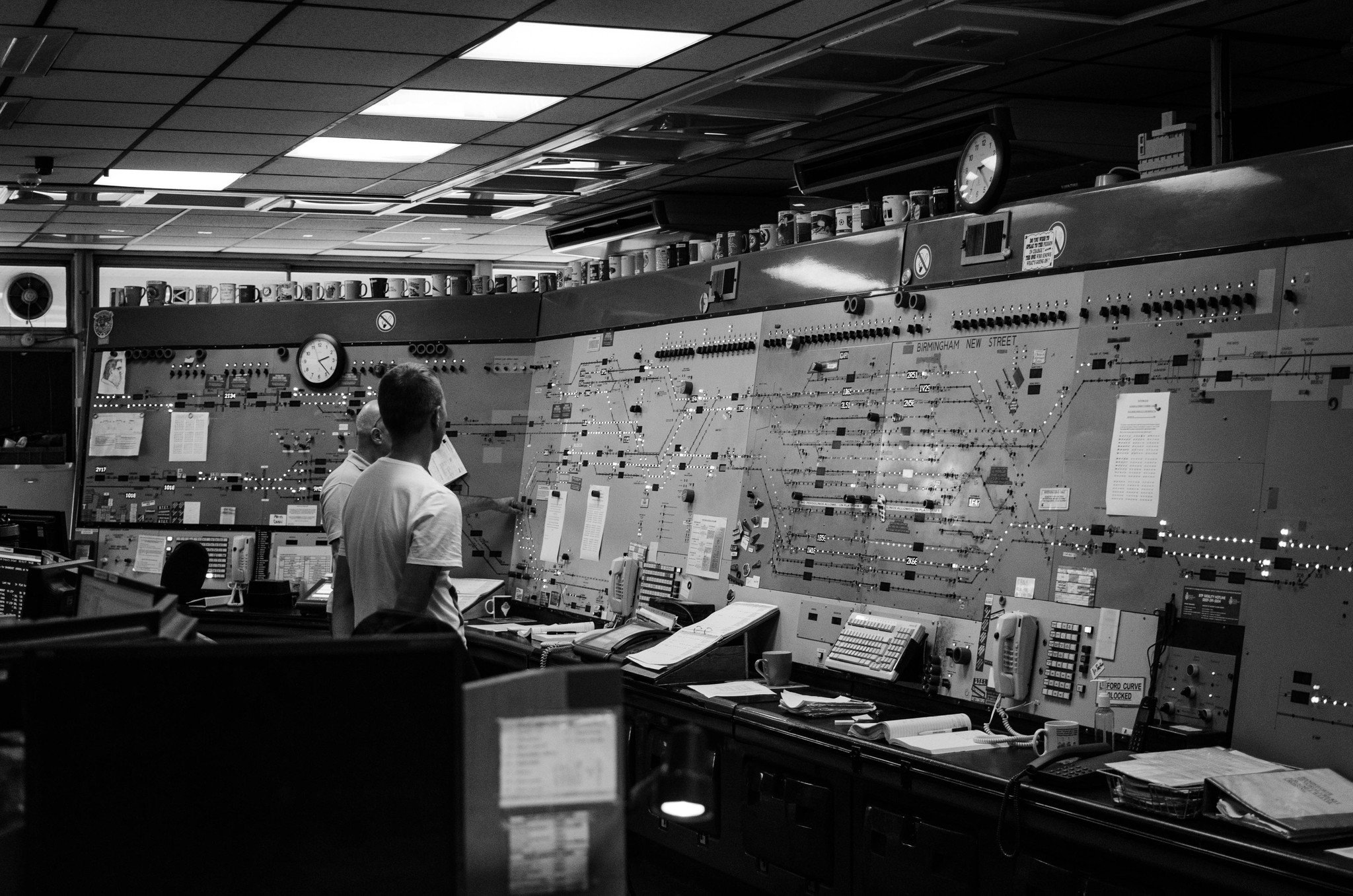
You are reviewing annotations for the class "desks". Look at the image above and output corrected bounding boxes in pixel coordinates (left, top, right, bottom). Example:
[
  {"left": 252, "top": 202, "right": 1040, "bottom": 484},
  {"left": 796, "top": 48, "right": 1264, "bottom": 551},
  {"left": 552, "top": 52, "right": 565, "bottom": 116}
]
[
  {"left": 733, "top": 694, "right": 1353, "bottom": 896},
  {"left": 464, "top": 618, "right": 856, "bottom": 894}
]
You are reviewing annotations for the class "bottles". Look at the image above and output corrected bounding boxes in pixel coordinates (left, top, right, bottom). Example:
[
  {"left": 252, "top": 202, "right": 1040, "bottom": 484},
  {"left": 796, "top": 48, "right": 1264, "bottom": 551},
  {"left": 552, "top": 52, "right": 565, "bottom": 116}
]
[{"left": 1090, "top": 679, "right": 1115, "bottom": 754}]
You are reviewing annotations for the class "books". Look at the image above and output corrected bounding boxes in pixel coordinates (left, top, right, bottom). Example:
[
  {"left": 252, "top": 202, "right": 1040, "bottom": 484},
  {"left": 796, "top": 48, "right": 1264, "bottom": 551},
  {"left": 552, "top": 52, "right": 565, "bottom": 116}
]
[
  {"left": 619, "top": 601, "right": 781, "bottom": 684},
  {"left": 845, "top": 713, "right": 1014, "bottom": 755}
]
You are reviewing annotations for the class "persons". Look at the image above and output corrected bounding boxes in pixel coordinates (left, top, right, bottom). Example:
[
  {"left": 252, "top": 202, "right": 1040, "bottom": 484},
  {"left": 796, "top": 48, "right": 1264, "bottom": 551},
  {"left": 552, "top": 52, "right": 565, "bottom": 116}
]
[
  {"left": 320, "top": 399, "right": 394, "bottom": 631},
  {"left": 330, "top": 363, "right": 467, "bottom": 661}
]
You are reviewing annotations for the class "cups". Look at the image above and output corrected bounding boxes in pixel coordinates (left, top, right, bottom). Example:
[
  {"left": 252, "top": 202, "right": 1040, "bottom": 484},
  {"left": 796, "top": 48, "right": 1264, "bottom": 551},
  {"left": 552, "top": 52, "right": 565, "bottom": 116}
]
[
  {"left": 556, "top": 178, "right": 966, "bottom": 294},
  {"left": 369, "top": 272, "right": 557, "bottom": 299},
  {"left": 755, "top": 651, "right": 793, "bottom": 686},
  {"left": 108, "top": 279, "right": 367, "bottom": 309},
  {"left": 1032, "top": 721, "right": 1079, "bottom": 757},
  {"left": 485, "top": 595, "right": 512, "bottom": 620}
]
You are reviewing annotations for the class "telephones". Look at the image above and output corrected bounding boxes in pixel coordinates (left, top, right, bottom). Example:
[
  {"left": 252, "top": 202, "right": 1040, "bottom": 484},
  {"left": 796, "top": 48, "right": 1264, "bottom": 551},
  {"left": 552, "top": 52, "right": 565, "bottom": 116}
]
[
  {"left": 1026, "top": 739, "right": 1139, "bottom": 786},
  {"left": 991, "top": 610, "right": 1039, "bottom": 701},
  {"left": 571, "top": 628, "right": 613, "bottom": 646},
  {"left": 607, "top": 555, "right": 638, "bottom": 616},
  {"left": 232, "top": 536, "right": 254, "bottom": 585},
  {"left": 608, "top": 628, "right": 674, "bottom": 664}
]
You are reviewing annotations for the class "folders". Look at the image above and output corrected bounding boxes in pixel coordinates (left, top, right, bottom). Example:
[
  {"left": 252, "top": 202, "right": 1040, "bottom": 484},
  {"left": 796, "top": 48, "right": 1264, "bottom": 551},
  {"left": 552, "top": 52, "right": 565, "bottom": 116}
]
[
  {"left": 1200, "top": 764, "right": 1353, "bottom": 844},
  {"left": 186, "top": 595, "right": 235, "bottom": 608}
]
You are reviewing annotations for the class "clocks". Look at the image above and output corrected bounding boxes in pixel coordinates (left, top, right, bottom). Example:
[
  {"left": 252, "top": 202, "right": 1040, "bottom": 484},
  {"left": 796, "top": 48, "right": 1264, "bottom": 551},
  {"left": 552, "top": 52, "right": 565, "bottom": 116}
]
[
  {"left": 955, "top": 124, "right": 1008, "bottom": 211},
  {"left": 296, "top": 333, "right": 346, "bottom": 391}
]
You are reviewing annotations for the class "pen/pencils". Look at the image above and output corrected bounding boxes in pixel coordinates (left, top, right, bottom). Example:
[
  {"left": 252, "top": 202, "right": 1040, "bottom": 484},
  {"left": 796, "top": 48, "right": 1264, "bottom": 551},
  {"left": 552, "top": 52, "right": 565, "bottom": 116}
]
[
  {"left": 543, "top": 631, "right": 579, "bottom": 635},
  {"left": 917, "top": 726, "right": 968, "bottom": 735}
]
